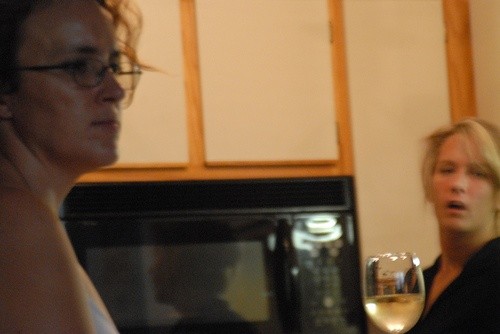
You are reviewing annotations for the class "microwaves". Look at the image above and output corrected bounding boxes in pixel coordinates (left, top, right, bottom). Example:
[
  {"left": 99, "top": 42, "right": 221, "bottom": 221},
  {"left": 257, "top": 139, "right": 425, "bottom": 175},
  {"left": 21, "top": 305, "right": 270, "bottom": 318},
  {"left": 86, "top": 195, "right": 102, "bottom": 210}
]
[{"left": 60, "top": 175, "right": 369, "bottom": 334}]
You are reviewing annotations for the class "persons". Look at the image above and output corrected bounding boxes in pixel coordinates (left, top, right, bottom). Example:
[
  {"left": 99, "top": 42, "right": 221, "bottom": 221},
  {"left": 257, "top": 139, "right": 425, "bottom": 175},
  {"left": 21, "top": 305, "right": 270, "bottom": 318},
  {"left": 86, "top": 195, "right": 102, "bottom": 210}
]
[
  {"left": 149, "top": 216, "right": 256, "bottom": 334},
  {"left": 401, "top": 117, "right": 500, "bottom": 334},
  {"left": 0, "top": 0, "right": 161, "bottom": 334}
]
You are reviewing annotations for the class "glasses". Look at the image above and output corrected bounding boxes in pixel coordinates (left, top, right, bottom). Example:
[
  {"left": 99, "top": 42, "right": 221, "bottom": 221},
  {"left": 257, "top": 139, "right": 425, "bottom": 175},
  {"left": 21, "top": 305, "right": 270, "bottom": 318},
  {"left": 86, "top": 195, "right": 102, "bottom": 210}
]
[{"left": 15, "top": 58, "right": 143, "bottom": 92}]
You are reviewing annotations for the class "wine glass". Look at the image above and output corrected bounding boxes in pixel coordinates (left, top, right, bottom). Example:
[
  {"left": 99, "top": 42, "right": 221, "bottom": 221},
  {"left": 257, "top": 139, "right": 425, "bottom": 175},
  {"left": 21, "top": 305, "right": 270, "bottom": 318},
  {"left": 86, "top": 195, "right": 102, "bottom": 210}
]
[{"left": 362, "top": 251, "right": 427, "bottom": 334}]
